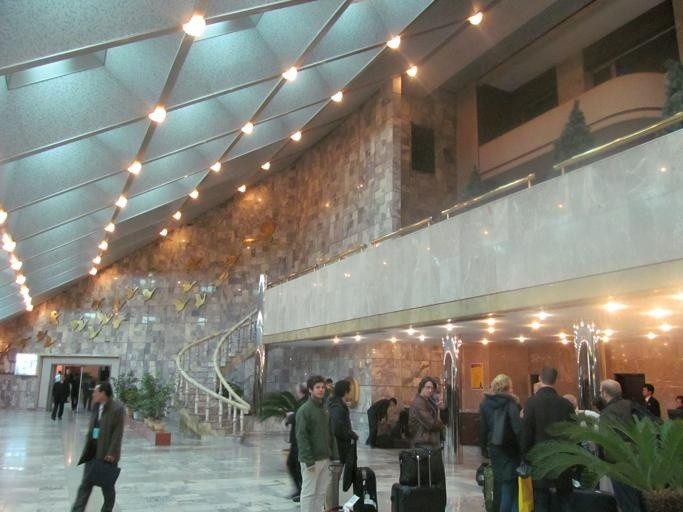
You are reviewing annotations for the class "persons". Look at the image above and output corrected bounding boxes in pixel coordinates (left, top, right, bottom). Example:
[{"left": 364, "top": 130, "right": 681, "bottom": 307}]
[
  {"left": 50, "top": 369, "right": 96, "bottom": 420},
  {"left": 363, "top": 398, "right": 398, "bottom": 449},
  {"left": 477, "top": 367, "right": 683, "bottom": 512},
  {"left": 283, "top": 376, "right": 358, "bottom": 512},
  {"left": 71, "top": 381, "right": 126, "bottom": 512},
  {"left": 408, "top": 378, "right": 449, "bottom": 451}
]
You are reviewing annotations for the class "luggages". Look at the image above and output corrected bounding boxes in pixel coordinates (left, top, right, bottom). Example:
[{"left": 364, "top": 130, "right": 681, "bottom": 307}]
[
  {"left": 390, "top": 449, "right": 447, "bottom": 512},
  {"left": 518, "top": 475, "right": 534, "bottom": 512},
  {"left": 551, "top": 488, "right": 617, "bottom": 512},
  {"left": 352, "top": 466, "right": 379, "bottom": 512}
]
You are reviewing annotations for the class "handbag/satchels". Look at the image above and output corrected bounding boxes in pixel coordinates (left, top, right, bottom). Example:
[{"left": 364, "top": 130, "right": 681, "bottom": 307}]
[
  {"left": 377, "top": 422, "right": 408, "bottom": 448},
  {"left": 484, "top": 465, "right": 500, "bottom": 511},
  {"left": 398, "top": 448, "right": 445, "bottom": 486},
  {"left": 83, "top": 459, "right": 121, "bottom": 489},
  {"left": 491, "top": 426, "right": 515, "bottom": 449},
  {"left": 632, "top": 401, "right": 665, "bottom": 444}
]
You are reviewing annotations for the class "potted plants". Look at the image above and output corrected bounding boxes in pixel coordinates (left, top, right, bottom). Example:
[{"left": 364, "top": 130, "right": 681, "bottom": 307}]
[
  {"left": 525, "top": 406, "right": 683, "bottom": 512},
  {"left": 115, "top": 370, "right": 174, "bottom": 429},
  {"left": 258, "top": 388, "right": 305, "bottom": 443}
]
